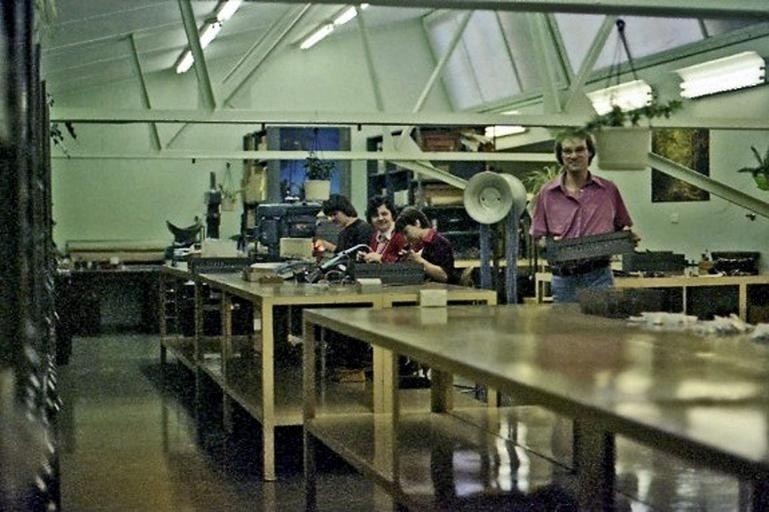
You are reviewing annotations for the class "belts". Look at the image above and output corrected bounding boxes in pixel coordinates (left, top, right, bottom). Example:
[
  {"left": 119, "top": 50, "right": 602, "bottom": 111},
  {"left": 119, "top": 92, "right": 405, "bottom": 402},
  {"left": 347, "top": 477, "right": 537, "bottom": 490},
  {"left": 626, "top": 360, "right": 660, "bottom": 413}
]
[{"left": 551, "top": 257, "right": 610, "bottom": 277}]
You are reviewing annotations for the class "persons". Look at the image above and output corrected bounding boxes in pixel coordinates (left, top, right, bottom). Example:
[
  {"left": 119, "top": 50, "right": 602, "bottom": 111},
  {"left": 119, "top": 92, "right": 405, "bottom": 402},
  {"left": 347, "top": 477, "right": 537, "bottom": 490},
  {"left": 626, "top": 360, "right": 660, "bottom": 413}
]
[
  {"left": 313, "top": 194, "right": 373, "bottom": 263},
  {"left": 393, "top": 204, "right": 455, "bottom": 286},
  {"left": 355, "top": 193, "right": 409, "bottom": 266},
  {"left": 528, "top": 131, "right": 643, "bottom": 303}
]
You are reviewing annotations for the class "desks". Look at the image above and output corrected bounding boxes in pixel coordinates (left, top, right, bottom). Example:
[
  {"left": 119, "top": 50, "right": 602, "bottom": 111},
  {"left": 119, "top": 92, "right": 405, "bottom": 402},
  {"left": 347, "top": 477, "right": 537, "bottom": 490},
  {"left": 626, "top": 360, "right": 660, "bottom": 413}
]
[
  {"left": 303, "top": 304, "right": 768, "bottom": 512},
  {"left": 160, "top": 258, "right": 497, "bottom": 479},
  {"left": 535, "top": 258, "right": 768, "bottom": 343},
  {"left": 452, "top": 259, "right": 604, "bottom": 303}
]
